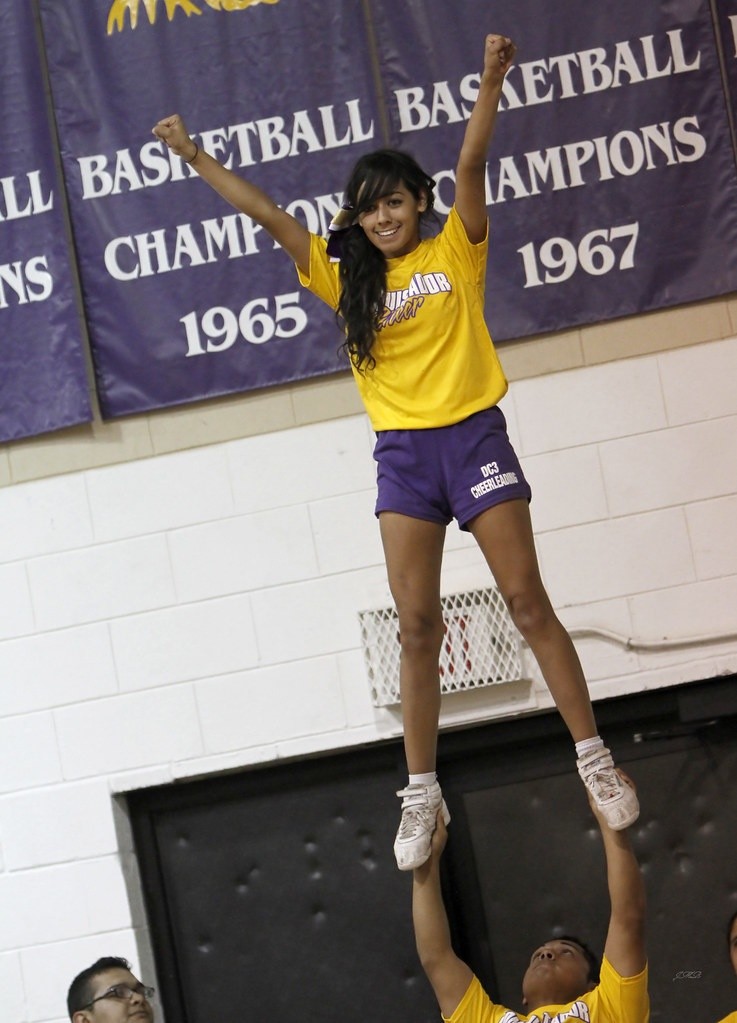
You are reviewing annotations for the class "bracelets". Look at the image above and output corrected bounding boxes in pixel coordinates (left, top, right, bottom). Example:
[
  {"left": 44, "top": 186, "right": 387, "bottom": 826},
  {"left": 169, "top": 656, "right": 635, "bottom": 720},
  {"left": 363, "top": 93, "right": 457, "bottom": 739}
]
[{"left": 184, "top": 142, "right": 199, "bottom": 162}]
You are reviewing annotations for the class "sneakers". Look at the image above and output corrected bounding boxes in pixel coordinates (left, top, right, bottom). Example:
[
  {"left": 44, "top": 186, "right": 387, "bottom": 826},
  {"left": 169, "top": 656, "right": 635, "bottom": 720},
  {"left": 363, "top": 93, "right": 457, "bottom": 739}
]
[
  {"left": 394, "top": 781, "right": 451, "bottom": 870},
  {"left": 576, "top": 745, "right": 640, "bottom": 831}
]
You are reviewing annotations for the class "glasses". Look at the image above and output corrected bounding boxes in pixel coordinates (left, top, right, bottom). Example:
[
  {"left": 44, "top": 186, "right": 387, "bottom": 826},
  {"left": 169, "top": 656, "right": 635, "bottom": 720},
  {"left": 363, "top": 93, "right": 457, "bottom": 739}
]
[{"left": 79, "top": 984, "right": 156, "bottom": 1011}]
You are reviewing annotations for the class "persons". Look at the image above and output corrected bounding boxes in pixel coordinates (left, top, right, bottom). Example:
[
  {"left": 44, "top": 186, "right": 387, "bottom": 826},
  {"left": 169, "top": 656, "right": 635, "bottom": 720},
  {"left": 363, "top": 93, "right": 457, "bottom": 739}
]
[
  {"left": 68, "top": 955, "right": 157, "bottom": 1023},
  {"left": 410, "top": 763, "right": 650, "bottom": 1023},
  {"left": 150, "top": 32, "right": 640, "bottom": 870},
  {"left": 716, "top": 915, "right": 737, "bottom": 1023}
]
